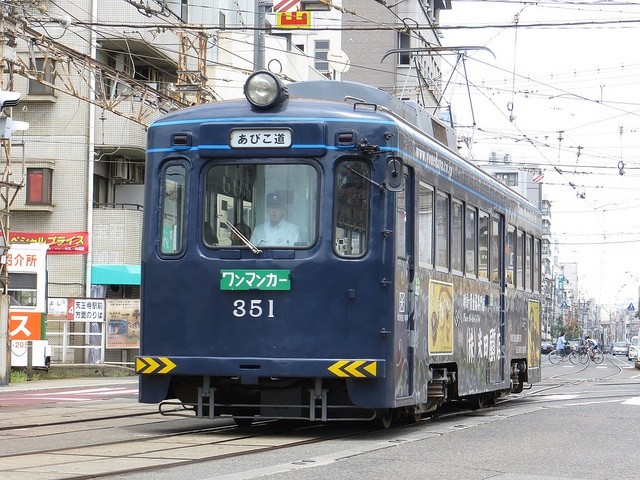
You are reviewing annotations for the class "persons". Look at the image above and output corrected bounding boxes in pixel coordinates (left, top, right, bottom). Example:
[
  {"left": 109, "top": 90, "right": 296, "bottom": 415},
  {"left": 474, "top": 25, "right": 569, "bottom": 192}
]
[
  {"left": 582, "top": 337, "right": 598, "bottom": 361},
  {"left": 555, "top": 332, "right": 571, "bottom": 363},
  {"left": 247, "top": 192, "right": 302, "bottom": 247}
]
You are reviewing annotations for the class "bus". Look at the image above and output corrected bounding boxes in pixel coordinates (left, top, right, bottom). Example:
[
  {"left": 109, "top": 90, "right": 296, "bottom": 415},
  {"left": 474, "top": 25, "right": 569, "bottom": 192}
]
[{"left": 134, "top": 70, "right": 544, "bottom": 433}]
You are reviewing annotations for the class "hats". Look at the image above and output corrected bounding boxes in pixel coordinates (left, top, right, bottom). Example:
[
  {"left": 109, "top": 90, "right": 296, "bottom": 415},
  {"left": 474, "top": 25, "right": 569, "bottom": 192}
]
[{"left": 266, "top": 193, "right": 286, "bottom": 207}]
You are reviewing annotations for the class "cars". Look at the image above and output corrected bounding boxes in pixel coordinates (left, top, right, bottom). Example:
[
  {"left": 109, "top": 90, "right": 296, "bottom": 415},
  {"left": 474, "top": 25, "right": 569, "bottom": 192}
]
[
  {"left": 612, "top": 341, "right": 629, "bottom": 356},
  {"left": 542, "top": 341, "right": 553, "bottom": 354}
]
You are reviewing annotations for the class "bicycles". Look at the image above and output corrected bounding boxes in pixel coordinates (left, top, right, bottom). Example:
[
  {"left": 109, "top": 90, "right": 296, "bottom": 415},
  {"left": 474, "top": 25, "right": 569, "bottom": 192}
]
[
  {"left": 548, "top": 345, "right": 583, "bottom": 365},
  {"left": 579, "top": 345, "right": 605, "bottom": 365}
]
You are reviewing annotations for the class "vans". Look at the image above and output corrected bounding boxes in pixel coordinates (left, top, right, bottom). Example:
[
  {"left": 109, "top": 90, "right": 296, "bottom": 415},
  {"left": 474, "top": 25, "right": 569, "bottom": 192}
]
[{"left": 628, "top": 336, "right": 639, "bottom": 361}]
